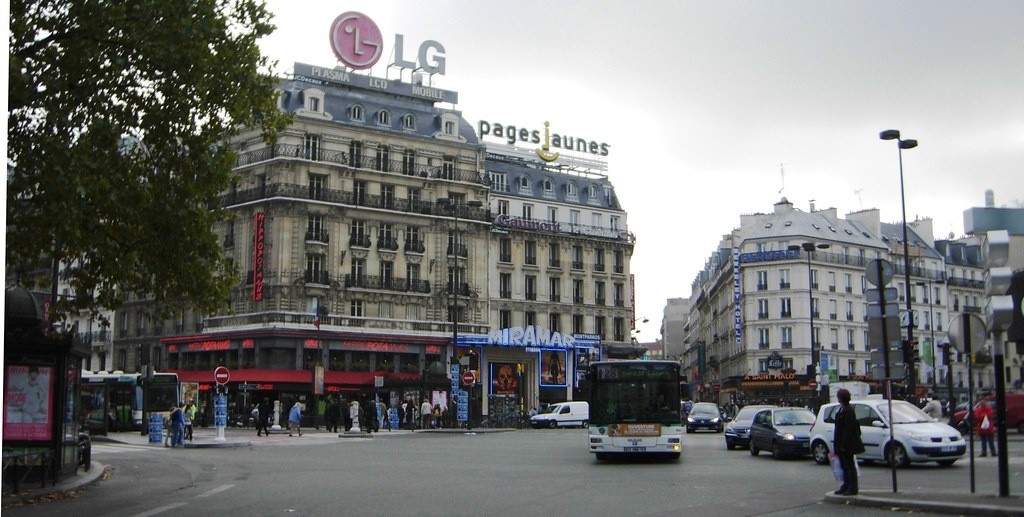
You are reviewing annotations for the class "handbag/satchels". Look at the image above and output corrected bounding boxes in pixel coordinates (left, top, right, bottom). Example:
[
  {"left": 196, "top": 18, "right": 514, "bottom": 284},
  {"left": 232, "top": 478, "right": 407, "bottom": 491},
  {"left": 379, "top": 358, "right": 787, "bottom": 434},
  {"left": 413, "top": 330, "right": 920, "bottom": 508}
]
[
  {"left": 981, "top": 416, "right": 989, "bottom": 429},
  {"left": 828, "top": 450, "right": 861, "bottom": 481}
]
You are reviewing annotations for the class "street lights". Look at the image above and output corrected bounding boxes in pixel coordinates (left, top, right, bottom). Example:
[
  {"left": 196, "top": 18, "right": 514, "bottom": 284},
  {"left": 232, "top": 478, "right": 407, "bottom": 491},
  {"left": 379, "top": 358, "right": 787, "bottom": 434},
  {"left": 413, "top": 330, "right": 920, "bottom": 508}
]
[
  {"left": 789, "top": 242, "right": 830, "bottom": 414},
  {"left": 880, "top": 129, "right": 918, "bottom": 409},
  {"left": 434, "top": 198, "right": 483, "bottom": 358}
]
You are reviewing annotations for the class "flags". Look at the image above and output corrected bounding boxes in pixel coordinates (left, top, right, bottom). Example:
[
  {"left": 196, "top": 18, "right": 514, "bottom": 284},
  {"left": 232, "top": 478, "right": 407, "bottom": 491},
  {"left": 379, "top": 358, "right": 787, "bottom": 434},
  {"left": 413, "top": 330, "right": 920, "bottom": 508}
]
[{"left": 314, "top": 303, "right": 319, "bottom": 328}]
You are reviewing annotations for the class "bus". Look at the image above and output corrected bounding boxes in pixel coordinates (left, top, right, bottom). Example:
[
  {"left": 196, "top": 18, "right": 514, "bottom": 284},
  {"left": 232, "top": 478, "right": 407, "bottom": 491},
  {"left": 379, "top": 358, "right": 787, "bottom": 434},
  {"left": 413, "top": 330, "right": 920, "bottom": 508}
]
[
  {"left": 578, "top": 358, "right": 690, "bottom": 458},
  {"left": 78, "top": 370, "right": 182, "bottom": 431}
]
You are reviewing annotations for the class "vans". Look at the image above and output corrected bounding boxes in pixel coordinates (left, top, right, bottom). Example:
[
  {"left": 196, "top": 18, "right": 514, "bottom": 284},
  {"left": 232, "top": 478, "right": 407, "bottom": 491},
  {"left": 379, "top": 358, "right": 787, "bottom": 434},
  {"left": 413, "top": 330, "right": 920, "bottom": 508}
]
[{"left": 530, "top": 400, "right": 589, "bottom": 429}]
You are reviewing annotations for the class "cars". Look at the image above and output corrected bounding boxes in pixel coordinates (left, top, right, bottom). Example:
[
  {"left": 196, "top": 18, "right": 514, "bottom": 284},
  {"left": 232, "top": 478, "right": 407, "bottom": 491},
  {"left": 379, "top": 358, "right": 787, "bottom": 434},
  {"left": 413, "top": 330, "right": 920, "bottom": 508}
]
[
  {"left": 724, "top": 404, "right": 818, "bottom": 459},
  {"left": 807, "top": 399, "right": 965, "bottom": 468},
  {"left": 686, "top": 402, "right": 727, "bottom": 433},
  {"left": 950, "top": 393, "right": 1024, "bottom": 433}
]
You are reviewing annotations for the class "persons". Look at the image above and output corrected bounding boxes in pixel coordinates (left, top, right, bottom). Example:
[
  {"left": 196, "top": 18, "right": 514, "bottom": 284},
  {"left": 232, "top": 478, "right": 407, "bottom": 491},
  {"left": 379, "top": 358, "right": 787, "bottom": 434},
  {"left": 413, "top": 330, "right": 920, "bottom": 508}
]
[
  {"left": 834, "top": 389, "right": 866, "bottom": 495},
  {"left": 680, "top": 399, "right": 694, "bottom": 427},
  {"left": 974, "top": 399, "right": 998, "bottom": 457},
  {"left": 288, "top": 402, "right": 303, "bottom": 437},
  {"left": 227, "top": 400, "right": 237, "bottom": 427},
  {"left": 921, "top": 394, "right": 942, "bottom": 422},
  {"left": 257, "top": 398, "right": 271, "bottom": 437},
  {"left": 397, "top": 396, "right": 450, "bottom": 430},
  {"left": 548, "top": 351, "right": 561, "bottom": 384},
  {"left": 199, "top": 400, "right": 211, "bottom": 427},
  {"left": 13, "top": 367, "right": 46, "bottom": 425},
  {"left": 324, "top": 398, "right": 386, "bottom": 433},
  {"left": 164, "top": 398, "right": 196, "bottom": 448}
]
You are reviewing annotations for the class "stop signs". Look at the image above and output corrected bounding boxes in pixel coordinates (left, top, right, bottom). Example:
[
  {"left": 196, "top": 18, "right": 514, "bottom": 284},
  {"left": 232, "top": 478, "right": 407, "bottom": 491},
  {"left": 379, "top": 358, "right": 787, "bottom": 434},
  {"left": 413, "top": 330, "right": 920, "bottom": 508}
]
[
  {"left": 462, "top": 372, "right": 474, "bottom": 384},
  {"left": 809, "top": 379, "right": 818, "bottom": 390},
  {"left": 214, "top": 366, "right": 230, "bottom": 384}
]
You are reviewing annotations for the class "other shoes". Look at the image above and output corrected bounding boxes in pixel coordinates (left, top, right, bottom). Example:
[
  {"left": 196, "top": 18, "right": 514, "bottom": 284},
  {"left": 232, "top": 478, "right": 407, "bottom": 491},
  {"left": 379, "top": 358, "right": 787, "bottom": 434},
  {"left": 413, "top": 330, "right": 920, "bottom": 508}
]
[
  {"left": 164, "top": 443, "right": 184, "bottom": 448},
  {"left": 834, "top": 489, "right": 843, "bottom": 494},
  {"left": 993, "top": 454, "right": 997, "bottom": 456},
  {"left": 843, "top": 491, "right": 856, "bottom": 495},
  {"left": 979, "top": 454, "right": 985, "bottom": 457}
]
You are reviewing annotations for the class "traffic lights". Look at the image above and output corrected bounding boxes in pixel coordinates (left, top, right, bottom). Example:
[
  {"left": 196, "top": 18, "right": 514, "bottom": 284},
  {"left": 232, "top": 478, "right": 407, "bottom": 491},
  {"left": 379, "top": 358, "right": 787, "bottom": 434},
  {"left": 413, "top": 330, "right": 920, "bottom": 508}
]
[
  {"left": 942, "top": 343, "right": 955, "bottom": 364},
  {"left": 909, "top": 340, "right": 921, "bottom": 365}
]
